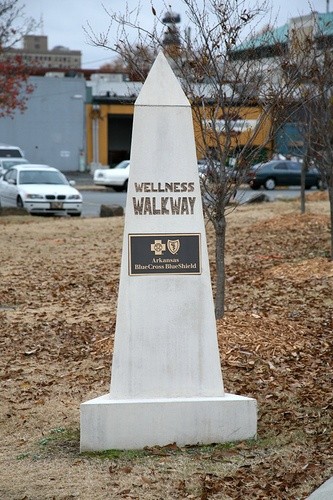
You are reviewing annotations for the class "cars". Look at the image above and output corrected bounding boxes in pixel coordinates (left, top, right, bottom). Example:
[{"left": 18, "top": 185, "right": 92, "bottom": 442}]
[
  {"left": 196, "top": 158, "right": 241, "bottom": 182},
  {"left": 0, "top": 144, "right": 24, "bottom": 158},
  {"left": 245, "top": 160, "right": 329, "bottom": 191},
  {"left": 94, "top": 160, "right": 207, "bottom": 192},
  {"left": 0, "top": 163, "right": 83, "bottom": 217},
  {"left": 1, "top": 156, "right": 29, "bottom": 178}
]
